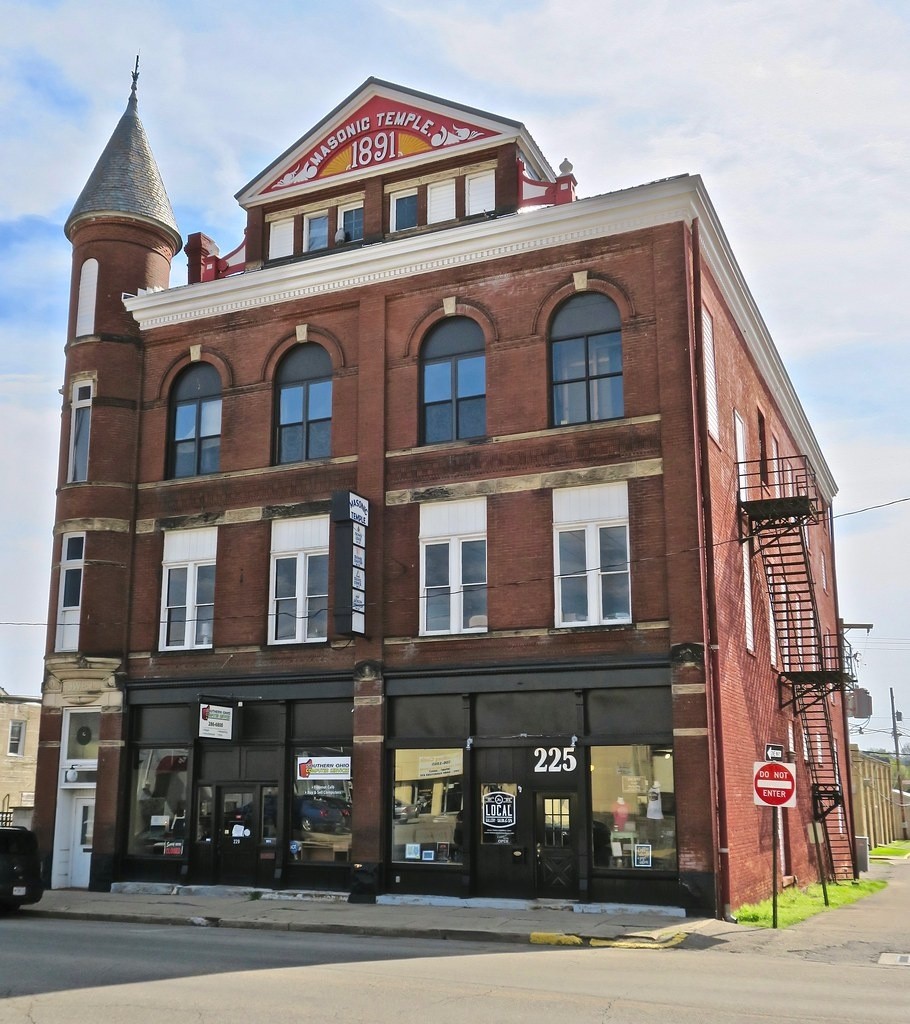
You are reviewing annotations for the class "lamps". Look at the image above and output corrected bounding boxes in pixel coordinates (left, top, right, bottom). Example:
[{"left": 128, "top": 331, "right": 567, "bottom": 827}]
[
  {"left": 67, "top": 764, "right": 80, "bottom": 782},
  {"left": 201, "top": 622, "right": 210, "bottom": 644}
]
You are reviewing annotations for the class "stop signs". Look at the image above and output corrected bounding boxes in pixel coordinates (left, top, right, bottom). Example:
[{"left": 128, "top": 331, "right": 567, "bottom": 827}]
[{"left": 753, "top": 761, "right": 796, "bottom": 807}]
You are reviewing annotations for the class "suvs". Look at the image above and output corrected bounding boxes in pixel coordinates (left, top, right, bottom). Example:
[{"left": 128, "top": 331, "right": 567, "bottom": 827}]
[{"left": 0, "top": 825, "right": 44, "bottom": 916}]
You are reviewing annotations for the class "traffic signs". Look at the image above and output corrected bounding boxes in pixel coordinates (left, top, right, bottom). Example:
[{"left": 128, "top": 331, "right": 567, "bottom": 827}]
[{"left": 764, "top": 743, "right": 783, "bottom": 762}]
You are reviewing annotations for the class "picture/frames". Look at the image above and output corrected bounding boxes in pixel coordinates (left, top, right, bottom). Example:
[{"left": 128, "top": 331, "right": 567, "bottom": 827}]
[{"left": 436, "top": 841, "right": 450, "bottom": 861}]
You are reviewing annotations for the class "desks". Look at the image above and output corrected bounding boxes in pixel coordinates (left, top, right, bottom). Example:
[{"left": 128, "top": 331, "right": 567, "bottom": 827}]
[{"left": 611, "top": 831, "right": 641, "bottom": 869}]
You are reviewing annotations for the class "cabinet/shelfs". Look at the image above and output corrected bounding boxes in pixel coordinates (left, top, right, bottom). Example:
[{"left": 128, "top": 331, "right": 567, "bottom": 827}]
[{"left": 294, "top": 840, "right": 350, "bottom": 863}]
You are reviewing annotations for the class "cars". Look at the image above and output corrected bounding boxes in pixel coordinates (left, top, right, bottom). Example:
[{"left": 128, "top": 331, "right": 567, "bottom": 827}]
[
  {"left": 395, "top": 798, "right": 420, "bottom": 825},
  {"left": 224, "top": 794, "right": 352, "bottom": 833}
]
